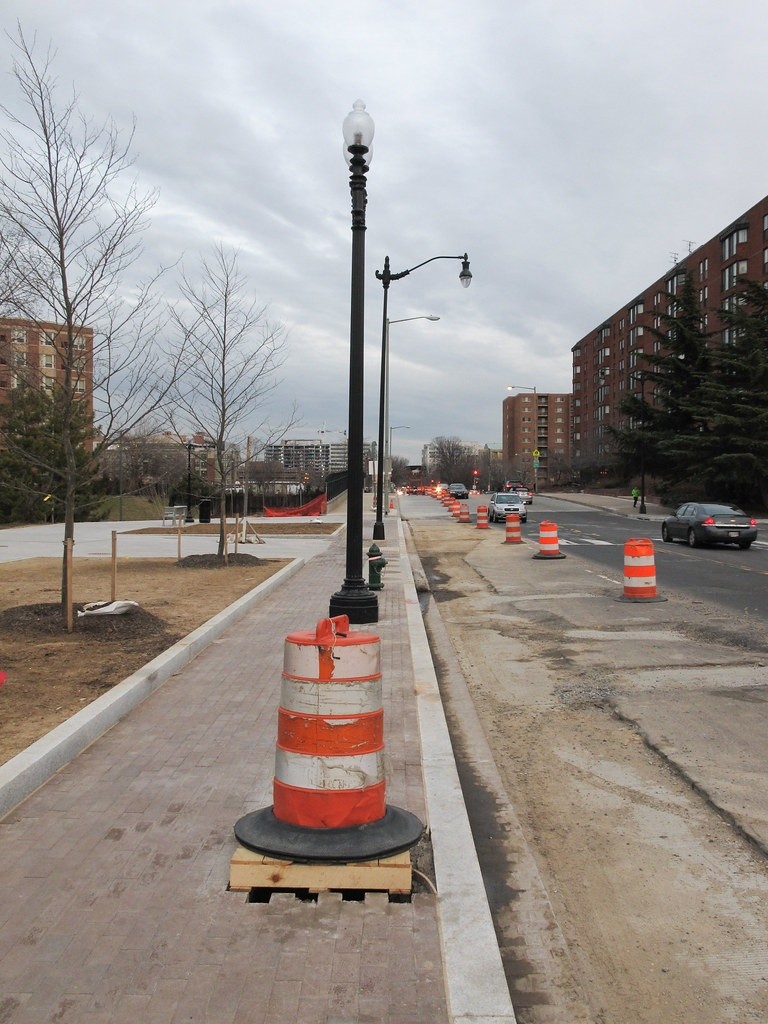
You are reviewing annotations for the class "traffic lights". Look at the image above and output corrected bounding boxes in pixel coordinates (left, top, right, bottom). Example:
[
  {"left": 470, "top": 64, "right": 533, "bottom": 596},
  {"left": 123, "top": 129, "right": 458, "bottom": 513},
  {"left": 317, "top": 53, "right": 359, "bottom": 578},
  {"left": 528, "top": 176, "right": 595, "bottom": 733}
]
[
  {"left": 474, "top": 468, "right": 478, "bottom": 477},
  {"left": 438, "top": 480, "right": 440, "bottom": 484},
  {"left": 431, "top": 479, "right": 434, "bottom": 483}
]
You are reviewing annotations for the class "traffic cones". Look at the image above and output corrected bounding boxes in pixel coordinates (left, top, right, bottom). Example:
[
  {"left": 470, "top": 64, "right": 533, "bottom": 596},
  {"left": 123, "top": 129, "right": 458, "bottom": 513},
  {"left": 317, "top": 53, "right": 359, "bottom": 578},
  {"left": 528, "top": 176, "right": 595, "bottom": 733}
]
[{"left": 389, "top": 499, "right": 395, "bottom": 509}]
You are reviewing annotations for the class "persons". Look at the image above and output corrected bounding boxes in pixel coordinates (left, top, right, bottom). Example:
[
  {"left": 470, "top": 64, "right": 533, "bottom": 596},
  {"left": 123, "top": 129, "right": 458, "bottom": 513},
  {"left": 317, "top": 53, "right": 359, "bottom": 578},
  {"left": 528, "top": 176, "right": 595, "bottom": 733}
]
[{"left": 631, "top": 487, "right": 640, "bottom": 507}]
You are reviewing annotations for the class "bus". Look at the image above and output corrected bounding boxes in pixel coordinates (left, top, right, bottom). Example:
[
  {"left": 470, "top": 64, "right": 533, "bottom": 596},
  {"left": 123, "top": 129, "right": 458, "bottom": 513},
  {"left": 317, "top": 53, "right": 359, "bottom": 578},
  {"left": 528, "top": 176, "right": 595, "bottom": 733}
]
[
  {"left": 164, "top": 505, "right": 189, "bottom": 521},
  {"left": 405, "top": 478, "right": 422, "bottom": 495}
]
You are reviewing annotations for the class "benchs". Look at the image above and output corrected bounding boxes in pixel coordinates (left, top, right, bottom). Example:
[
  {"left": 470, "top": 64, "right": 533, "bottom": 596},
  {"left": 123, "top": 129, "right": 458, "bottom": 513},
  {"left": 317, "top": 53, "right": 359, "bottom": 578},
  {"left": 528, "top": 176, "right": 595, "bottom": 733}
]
[{"left": 162, "top": 505, "right": 187, "bottom": 527}]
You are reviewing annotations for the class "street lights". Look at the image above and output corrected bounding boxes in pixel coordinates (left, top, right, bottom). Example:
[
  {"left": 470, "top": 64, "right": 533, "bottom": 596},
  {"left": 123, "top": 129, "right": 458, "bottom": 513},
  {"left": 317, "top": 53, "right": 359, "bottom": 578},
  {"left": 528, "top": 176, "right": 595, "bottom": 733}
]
[
  {"left": 374, "top": 253, "right": 472, "bottom": 541},
  {"left": 390, "top": 426, "right": 411, "bottom": 456},
  {"left": 600, "top": 369, "right": 647, "bottom": 514},
  {"left": 506, "top": 386, "right": 537, "bottom": 457},
  {"left": 328, "top": 98, "right": 379, "bottom": 628},
  {"left": 185, "top": 444, "right": 215, "bottom": 522},
  {"left": 385, "top": 316, "right": 440, "bottom": 511}
]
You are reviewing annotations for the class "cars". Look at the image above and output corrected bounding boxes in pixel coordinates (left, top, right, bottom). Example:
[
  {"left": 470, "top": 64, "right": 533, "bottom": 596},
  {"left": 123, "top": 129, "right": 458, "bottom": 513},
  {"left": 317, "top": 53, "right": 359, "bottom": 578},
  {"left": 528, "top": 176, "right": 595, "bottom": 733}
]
[
  {"left": 503, "top": 480, "right": 524, "bottom": 493},
  {"left": 487, "top": 492, "right": 527, "bottom": 523},
  {"left": 662, "top": 501, "right": 758, "bottom": 549},
  {"left": 509, "top": 487, "right": 533, "bottom": 504},
  {"left": 447, "top": 483, "right": 469, "bottom": 499},
  {"left": 437, "top": 483, "right": 449, "bottom": 493}
]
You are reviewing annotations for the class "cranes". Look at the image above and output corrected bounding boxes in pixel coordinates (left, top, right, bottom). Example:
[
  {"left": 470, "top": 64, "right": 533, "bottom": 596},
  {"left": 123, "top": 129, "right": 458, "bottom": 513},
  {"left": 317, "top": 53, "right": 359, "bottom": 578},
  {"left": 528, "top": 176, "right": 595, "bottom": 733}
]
[{"left": 316, "top": 421, "right": 348, "bottom": 444}]
